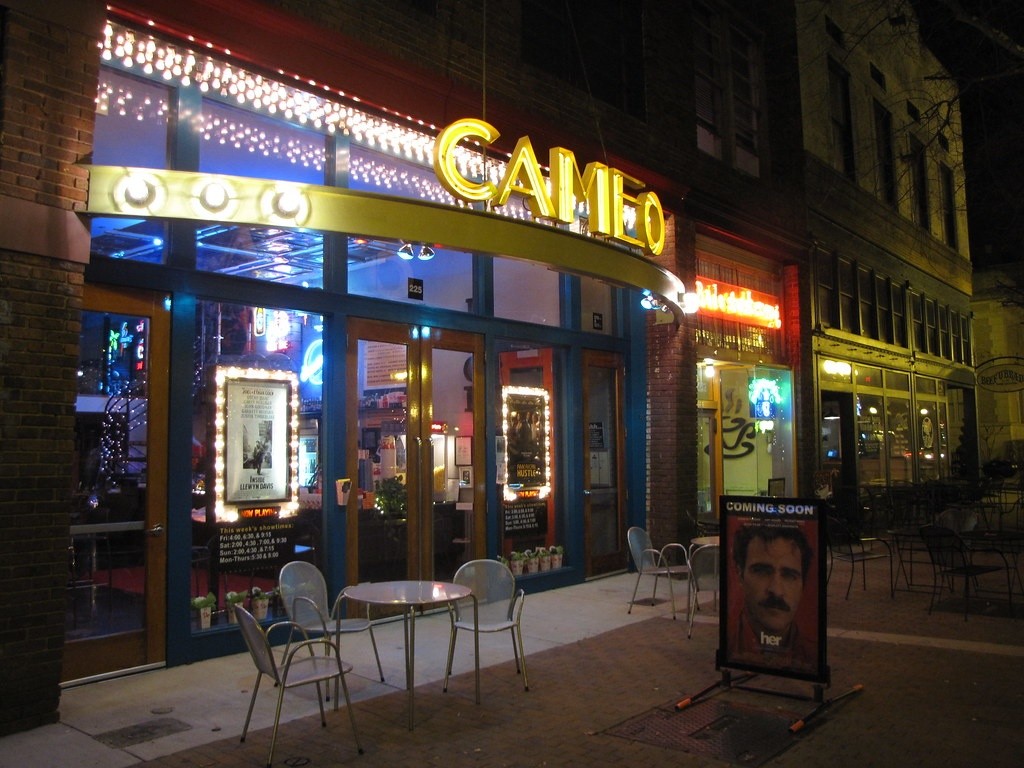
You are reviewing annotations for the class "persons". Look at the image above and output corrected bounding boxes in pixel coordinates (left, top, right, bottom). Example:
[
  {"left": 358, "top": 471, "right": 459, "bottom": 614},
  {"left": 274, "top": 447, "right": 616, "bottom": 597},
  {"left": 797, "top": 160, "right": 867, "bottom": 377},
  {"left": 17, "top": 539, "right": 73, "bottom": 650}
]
[
  {"left": 253, "top": 440, "right": 266, "bottom": 476},
  {"left": 510, "top": 409, "right": 543, "bottom": 442},
  {"left": 75, "top": 429, "right": 124, "bottom": 491},
  {"left": 730, "top": 521, "right": 816, "bottom": 671}
]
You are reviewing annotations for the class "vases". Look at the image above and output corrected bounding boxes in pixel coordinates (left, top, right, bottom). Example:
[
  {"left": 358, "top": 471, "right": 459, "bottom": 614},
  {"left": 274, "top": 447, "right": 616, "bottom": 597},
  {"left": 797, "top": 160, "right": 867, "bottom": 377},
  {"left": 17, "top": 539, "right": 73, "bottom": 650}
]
[
  {"left": 540, "top": 556, "right": 551, "bottom": 571},
  {"left": 528, "top": 559, "right": 538, "bottom": 573},
  {"left": 226, "top": 604, "right": 243, "bottom": 624},
  {"left": 198, "top": 607, "right": 212, "bottom": 629},
  {"left": 510, "top": 560, "right": 523, "bottom": 576},
  {"left": 251, "top": 599, "right": 269, "bottom": 620},
  {"left": 551, "top": 554, "right": 562, "bottom": 569}
]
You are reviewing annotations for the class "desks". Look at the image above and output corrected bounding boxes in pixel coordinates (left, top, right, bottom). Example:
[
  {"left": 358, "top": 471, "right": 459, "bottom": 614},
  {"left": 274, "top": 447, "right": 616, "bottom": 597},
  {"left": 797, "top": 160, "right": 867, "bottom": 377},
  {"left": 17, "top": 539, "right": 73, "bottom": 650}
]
[
  {"left": 325, "top": 581, "right": 480, "bottom": 732},
  {"left": 887, "top": 528, "right": 956, "bottom": 595},
  {"left": 683, "top": 536, "right": 720, "bottom": 612},
  {"left": 959, "top": 530, "right": 1024, "bottom": 617}
]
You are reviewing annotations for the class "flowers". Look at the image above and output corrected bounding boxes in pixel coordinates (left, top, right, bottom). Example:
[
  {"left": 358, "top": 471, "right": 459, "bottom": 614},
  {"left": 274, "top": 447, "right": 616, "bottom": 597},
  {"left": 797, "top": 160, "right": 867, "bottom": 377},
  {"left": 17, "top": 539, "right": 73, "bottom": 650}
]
[
  {"left": 225, "top": 590, "right": 248, "bottom": 608},
  {"left": 535, "top": 546, "right": 550, "bottom": 557},
  {"left": 509, "top": 550, "right": 524, "bottom": 560},
  {"left": 524, "top": 550, "right": 538, "bottom": 559},
  {"left": 251, "top": 587, "right": 268, "bottom": 601},
  {"left": 549, "top": 545, "right": 564, "bottom": 554},
  {"left": 497, "top": 556, "right": 510, "bottom": 568},
  {"left": 191, "top": 592, "right": 217, "bottom": 612}
]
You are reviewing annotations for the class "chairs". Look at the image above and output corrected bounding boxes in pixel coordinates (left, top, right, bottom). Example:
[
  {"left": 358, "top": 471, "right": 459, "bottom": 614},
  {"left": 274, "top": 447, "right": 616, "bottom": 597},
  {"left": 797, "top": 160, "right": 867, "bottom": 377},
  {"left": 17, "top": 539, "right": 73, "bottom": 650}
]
[
  {"left": 626, "top": 527, "right": 701, "bottom": 621},
  {"left": 825, "top": 460, "right": 1024, "bottom": 616},
  {"left": 272, "top": 560, "right": 385, "bottom": 702},
  {"left": 442, "top": 559, "right": 529, "bottom": 692},
  {"left": 688, "top": 544, "right": 719, "bottom": 638},
  {"left": 233, "top": 603, "right": 364, "bottom": 768}
]
